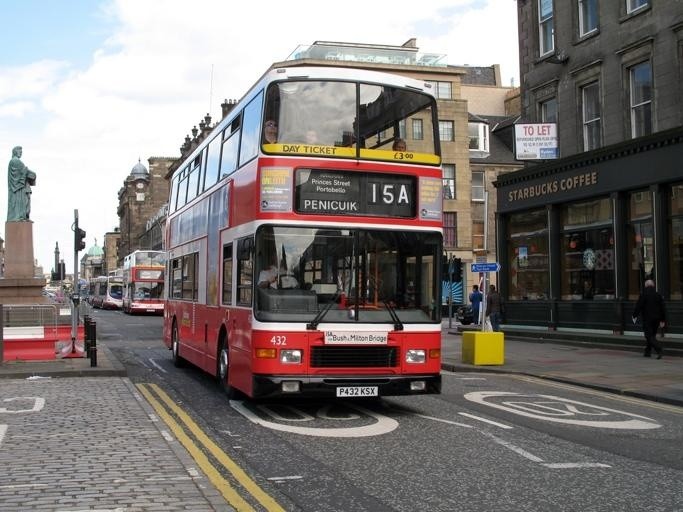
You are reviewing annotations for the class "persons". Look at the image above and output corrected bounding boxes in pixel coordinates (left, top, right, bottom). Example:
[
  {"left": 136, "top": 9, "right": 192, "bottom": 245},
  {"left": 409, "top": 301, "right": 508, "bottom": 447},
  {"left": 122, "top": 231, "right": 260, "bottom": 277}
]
[
  {"left": 469, "top": 284, "right": 483, "bottom": 325},
  {"left": 633, "top": 280, "right": 666, "bottom": 360},
  {"left": 306, "top": 128, "right": 334, "bottom": 146},
  {"left": 392, "top": 137, "right": 406, "bottom": 151},
  {"left": 257, "top": 245, "right": 299, "bottom": 289},
  {"left": 6, "top": 146, "right": 36, "bottom": 222},
  {"left": 486, "top": 285, "right": 506, "bottom": 332},
  {"left": 262, "top": 120, "right": 279, "bottom": 144}
]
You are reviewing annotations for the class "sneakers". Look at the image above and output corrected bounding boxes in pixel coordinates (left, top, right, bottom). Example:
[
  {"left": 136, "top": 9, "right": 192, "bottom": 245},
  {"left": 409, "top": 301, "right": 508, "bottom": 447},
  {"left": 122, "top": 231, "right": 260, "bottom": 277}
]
[{"left": 643, "top": 346, "right": 665, "bottom": 361}]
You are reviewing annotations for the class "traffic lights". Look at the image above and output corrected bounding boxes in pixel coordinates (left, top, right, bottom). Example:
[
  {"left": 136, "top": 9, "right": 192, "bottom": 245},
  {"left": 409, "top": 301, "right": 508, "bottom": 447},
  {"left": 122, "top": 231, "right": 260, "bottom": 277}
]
[{"left": 75, "top": 227, "right": 85, "bottom": 251}]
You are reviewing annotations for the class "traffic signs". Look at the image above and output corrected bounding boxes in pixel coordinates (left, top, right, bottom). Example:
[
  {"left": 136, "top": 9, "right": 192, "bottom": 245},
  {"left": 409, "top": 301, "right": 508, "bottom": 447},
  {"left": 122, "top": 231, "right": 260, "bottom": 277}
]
[{"left": 470, "top": 262, "right": 499, "bottom": 273}]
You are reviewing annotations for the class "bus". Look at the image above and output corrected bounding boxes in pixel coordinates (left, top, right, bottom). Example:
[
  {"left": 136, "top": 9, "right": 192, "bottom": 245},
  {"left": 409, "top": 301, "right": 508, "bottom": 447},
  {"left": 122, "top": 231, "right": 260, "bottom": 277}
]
[
  {"left": 86, "top": 273, "right": 123, "bottom": 311},
  {"left": 508, "top": 210, "right": 681, "bottom": 299},
  {"left": 120, "top": 249, "right": 166, "bottom": 318},
  {"left": 163, "top": 64, "right": 448, "bottom": 398}
]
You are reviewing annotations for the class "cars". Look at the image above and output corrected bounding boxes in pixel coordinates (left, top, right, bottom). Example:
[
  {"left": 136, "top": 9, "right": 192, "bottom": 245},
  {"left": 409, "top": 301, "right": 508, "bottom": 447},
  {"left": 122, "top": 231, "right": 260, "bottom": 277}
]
[{"left": 454, "top": 303, "right": 474, "bottom": 325}]
